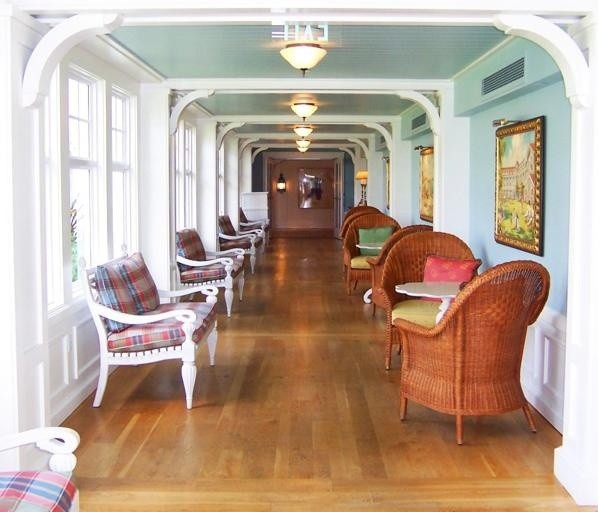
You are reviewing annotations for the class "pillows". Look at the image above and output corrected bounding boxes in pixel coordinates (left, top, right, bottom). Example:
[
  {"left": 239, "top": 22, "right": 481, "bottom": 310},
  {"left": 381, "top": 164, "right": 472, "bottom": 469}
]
[
  {"left": 356, "top": 226, "right": 393, "bottom": 255},
  {"left": 420, "top": 253, "right": 482, "bottom": 305}
]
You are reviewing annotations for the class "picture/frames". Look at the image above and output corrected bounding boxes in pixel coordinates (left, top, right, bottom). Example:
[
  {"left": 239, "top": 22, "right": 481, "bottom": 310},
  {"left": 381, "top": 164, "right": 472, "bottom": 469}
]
[
  {"left": 420, "top": 147, "right": 433, "bottom": 223},
  {"left": 495, "top": 115, "right": 545, "bottom": 257},
  {"left": 386, "top": 159, "right": 390, "bottom": 209}
]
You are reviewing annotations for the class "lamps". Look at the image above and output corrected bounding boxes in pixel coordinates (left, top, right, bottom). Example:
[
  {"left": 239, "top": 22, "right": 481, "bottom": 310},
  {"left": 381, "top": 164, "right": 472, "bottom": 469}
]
[
  {"left": 279, "top": 43, "right": 327, "bottom": 77},
  {"left": 276, "top": 176, "right": 286, "bottom": 193},
  {"left": 290, "top": 102, "right": 318, "bottom": 154},
  {"left": 355, "top": 170, "right": 368, "bottom": 206}
]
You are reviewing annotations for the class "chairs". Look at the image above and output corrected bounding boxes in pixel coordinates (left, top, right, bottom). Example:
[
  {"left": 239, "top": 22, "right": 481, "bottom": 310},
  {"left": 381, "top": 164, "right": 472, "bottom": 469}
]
[
  {"left": 87, "top": 208, "right": 270, "bottom": 409},
  {"left": 365, "top": 223, "right": 432, "bottom": 316},
  {"left": 392, "top": 257, "right": 549, "bottom": 446},
  {"left": 0, "top": 427, "right": 80, "bottom": 512},
  {"left": 376, "top": 231, "right": 479, "bottom": 371},
  {"left": 341, "top": 206, "right": 402, "bottom": 294}
]
